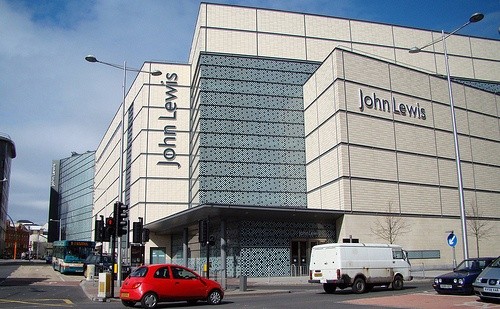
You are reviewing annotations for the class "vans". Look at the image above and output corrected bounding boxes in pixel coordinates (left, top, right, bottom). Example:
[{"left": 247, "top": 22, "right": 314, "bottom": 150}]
[{"left": 307, "top": 242, "right": 414, "bottom": 294}]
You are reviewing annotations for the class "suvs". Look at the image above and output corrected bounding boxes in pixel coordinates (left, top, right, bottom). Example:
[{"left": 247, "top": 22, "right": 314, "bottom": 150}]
[{"left": 83, "top": 253, "right": 131, "bottom": 278}]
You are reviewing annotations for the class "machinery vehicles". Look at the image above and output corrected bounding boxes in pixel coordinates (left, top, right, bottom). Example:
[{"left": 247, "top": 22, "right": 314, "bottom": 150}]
[{"left": 21, "top": 251, "right": 32, "bottom": 260}]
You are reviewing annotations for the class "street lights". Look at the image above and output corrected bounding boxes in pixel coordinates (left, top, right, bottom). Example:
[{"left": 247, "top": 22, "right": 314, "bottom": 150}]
[
  {"left": 84, "top": 55, "right": 164, "bottom": 296},
  {"left": 49, "top": 219, "right": 62, "bottom": 241},
  {"left": 409, "top": 11, "right": 486, "bottom": 269}
]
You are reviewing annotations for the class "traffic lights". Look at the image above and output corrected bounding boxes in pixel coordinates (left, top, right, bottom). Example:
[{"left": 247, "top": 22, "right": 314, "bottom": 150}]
[
  {"left": 135, "top": 222, "right": 140, "bottom": 242},
  {"left": 96, "top": 221, "right": 103, "bottom": 241},
  {"left": 106, "top": 217, "right": 114, "bottom": 235},
  {"left": 116, "top": 202, "right": 128, "bottom": 237}
]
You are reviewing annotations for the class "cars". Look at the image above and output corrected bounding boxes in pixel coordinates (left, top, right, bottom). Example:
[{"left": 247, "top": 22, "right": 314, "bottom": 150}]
[
  {"left": 41, "top": 255, "right": 49, "bottom": 260},
  {"left": 432, "top": 257, "right": 497, "bottom": 295},
  {"left": 119, "top": 264, "right": 225, "bottom": 308},
  {"left": 472, "top": 256, "right": 500, "bottom": 303},
  {"left": 32, "top": 253, "right": 42, "bottom": 260},
  {"left": 46, "top": 257, "right": 52, "bottom": 263}
]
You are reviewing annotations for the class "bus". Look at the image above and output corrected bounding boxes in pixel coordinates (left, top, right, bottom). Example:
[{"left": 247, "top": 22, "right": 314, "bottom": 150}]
[{"left": 53, "top": 239, "right": 98, "bottom": 276}]
